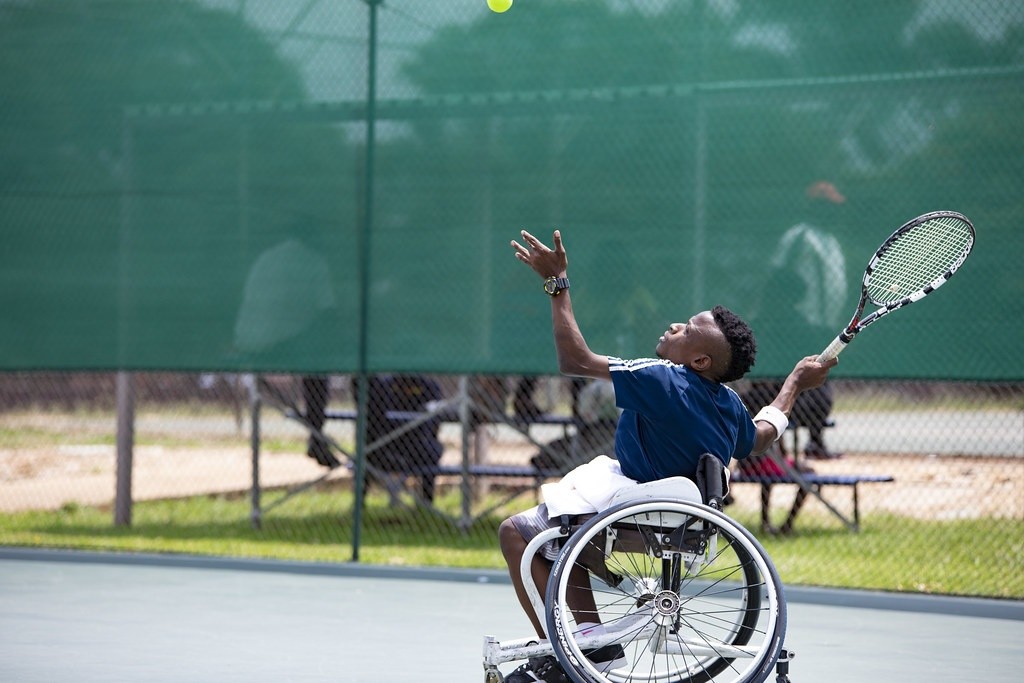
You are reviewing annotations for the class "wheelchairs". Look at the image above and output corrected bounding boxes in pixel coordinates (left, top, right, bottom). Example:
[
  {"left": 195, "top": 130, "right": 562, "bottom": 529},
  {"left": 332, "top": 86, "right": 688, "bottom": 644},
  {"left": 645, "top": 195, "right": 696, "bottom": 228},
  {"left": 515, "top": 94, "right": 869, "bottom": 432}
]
[{"left": 482, "top": 451, "right": 797, "bottom": 683}]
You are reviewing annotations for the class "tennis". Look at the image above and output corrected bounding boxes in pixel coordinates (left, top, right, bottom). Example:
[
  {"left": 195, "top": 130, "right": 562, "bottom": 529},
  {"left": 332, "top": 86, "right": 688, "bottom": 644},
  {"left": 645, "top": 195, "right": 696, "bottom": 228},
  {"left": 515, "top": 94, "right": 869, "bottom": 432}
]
[{"left": 485, "top": 0, "right": 512, "bottom": 14}]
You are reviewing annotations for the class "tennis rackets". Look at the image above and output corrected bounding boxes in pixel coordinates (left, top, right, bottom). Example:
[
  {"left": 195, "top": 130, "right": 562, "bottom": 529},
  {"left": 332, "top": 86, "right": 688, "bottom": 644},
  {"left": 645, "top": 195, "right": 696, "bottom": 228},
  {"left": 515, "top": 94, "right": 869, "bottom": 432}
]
[{"left": 814, "top": 209, "right": 976, "bottom": 364}]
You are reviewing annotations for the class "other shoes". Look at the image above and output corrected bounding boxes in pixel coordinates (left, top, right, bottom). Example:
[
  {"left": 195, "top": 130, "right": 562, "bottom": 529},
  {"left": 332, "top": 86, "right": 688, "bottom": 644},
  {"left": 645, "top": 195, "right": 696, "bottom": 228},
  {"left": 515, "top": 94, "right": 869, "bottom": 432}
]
[
  {"left": 582, "top": 643, "right": 627, "bottom": 671},
  {"left": 508, "top": 663, "right": 548, "bottom": 682}
]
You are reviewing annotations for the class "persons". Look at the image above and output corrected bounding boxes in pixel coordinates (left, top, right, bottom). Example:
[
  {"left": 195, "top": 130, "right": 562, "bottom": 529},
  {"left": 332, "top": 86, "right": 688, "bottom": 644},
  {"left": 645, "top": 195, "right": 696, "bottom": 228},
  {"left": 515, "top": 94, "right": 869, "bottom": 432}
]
[
  {"left": 501, "top": 229, "right": 840, "bottom": 682},
  {"left": 294, "top": 373, "right": 838, "bottom": 520}
]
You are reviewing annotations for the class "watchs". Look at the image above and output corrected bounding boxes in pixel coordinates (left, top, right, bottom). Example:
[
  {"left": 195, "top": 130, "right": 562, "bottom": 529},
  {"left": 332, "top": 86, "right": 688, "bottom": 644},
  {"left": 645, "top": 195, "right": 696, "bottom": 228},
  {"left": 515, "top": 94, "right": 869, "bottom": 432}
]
[{"left": 543, "top": 275, "right": 570, "bottom": 297}]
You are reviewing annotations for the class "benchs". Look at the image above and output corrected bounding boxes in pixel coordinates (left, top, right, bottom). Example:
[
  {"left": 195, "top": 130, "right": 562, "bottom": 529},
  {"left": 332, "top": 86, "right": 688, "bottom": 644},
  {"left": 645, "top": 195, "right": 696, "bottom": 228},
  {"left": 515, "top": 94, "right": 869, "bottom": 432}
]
[{"left": 290, "top": 410, "right": 895, "bottom": 538}]
